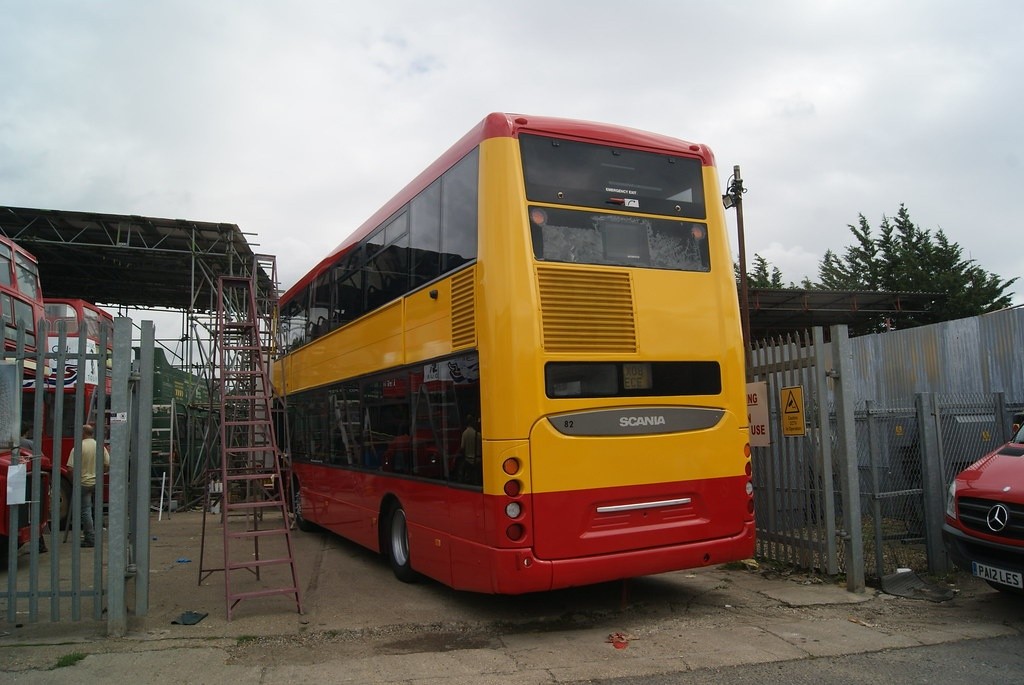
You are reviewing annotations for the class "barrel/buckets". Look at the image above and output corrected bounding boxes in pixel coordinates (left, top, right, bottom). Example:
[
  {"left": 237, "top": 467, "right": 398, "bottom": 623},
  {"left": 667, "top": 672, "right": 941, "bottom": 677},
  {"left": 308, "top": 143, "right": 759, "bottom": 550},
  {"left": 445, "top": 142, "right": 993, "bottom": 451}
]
[{"left": 211, "top": 499, "right": 220, "bottom": 513}]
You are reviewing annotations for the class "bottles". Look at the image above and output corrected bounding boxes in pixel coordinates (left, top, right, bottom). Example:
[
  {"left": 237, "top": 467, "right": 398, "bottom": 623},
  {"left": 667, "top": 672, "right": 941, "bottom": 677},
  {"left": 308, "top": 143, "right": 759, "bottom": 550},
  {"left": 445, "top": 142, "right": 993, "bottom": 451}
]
[{"left": 208, "top": 477, "right": 223, "bottom": 492}]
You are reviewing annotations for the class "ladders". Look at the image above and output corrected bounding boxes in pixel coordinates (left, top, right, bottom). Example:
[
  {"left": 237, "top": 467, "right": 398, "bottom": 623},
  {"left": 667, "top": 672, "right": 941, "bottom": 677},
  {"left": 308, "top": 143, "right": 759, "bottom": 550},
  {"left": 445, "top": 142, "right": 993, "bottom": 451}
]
[
  {"left": 61, "top": 385, "right": 111, "bottom": 543},
  {"left": 197, "top": 274, "right": 304, "bottom": 623},
  {"left": 415, "top": 359, "right": 464, "bottom": 483},
  {"left": 219, "top": 253, "right": 299, "bottom": 534},
  {"left": 150, "top": 396, "right": 187, "bottom": 520},
  {"left": 328, "top": 393, "right": 379, "bottom": 469}
]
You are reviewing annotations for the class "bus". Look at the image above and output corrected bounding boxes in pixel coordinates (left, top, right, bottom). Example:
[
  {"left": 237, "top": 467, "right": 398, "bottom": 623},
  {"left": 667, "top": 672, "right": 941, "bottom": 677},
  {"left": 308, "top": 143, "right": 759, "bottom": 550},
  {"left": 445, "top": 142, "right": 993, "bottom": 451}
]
[
  {"left": 20, "top": 296, "right": 115, "bottom": 531},
  {"left": 356, "top": 358, "right": 481, "bottom": 481},
  {"left": 0, "top": 234, "right": 51, "bottom": 569},
  {"left": 274, "top": 110, "right": 757, "bottom": 597}
]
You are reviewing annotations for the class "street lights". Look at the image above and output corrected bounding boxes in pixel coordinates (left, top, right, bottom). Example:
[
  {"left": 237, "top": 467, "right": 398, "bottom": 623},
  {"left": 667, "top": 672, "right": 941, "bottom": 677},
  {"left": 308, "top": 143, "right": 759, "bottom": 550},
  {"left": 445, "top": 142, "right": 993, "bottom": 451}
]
[{"left": 722, "top": 164, "right": 755, "bottom": 382}]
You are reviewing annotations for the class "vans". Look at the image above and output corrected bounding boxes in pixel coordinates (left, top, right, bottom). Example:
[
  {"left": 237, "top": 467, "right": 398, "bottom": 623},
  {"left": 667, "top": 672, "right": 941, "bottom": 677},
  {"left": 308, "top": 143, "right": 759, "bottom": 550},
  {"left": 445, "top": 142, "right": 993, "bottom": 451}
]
[{"left": 941, "top": 421, "right": 1024, "bottom": 597}]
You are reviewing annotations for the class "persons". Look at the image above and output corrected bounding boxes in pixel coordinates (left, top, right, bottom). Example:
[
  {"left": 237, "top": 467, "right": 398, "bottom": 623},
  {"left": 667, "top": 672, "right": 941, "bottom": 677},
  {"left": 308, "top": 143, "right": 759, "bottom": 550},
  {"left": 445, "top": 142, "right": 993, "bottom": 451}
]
[{"left": 65, "top": 421, "right": 112, "bottom": 547}]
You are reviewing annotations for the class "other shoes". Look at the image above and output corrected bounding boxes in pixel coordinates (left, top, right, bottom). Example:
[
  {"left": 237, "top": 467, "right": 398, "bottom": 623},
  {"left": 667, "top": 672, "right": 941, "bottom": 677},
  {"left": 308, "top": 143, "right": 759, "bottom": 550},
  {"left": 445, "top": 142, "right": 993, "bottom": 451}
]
[
  {"left": 39, "top": 548, "right": 48, "bottom": 553},
  {"left": 81, "top": 541, "right": 94, "bottom": 547}
]
